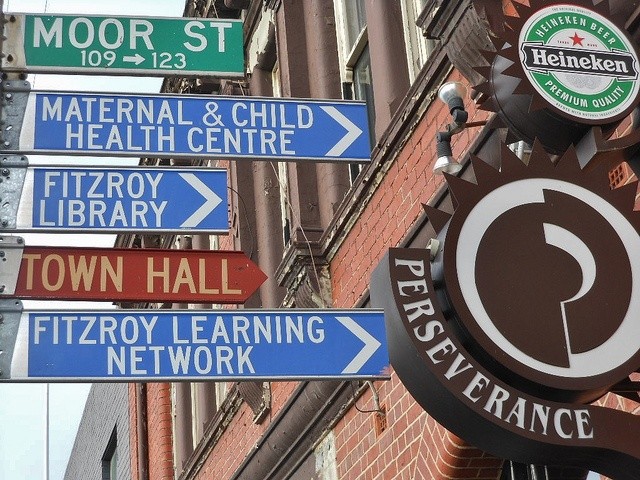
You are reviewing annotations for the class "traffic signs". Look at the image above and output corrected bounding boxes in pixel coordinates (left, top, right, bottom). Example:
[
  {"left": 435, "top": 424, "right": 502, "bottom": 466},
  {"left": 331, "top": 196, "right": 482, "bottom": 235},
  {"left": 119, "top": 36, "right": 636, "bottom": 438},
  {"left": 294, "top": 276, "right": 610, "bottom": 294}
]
[
  {"left": 0, "top": 246, "right": 268, "bottom": 306},
  {"left": 2, "top": 12, "right": 246, "bottom": 78},
  {"left": 0, "top": 308, "right": 392, "bottom": 381},
  {"left": 0, "top": 164, "right": 228, "bottom": 236},
  {"left": 0, "top": 86, "right": 373, "bottom": 164}
]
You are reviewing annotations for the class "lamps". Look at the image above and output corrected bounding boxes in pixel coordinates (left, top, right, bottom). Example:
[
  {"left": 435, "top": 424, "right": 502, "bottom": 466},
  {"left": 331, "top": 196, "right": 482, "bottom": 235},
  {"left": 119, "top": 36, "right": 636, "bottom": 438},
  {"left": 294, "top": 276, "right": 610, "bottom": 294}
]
[{"left": 429, "top": 85, "right": 489, "bottom": 178}]
